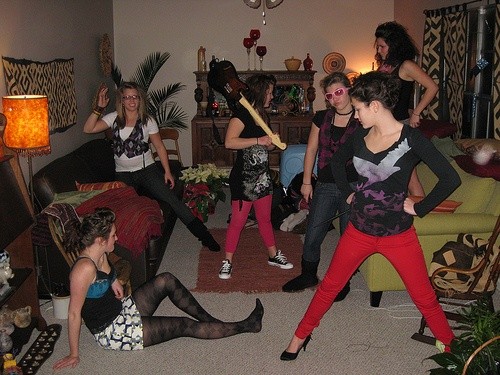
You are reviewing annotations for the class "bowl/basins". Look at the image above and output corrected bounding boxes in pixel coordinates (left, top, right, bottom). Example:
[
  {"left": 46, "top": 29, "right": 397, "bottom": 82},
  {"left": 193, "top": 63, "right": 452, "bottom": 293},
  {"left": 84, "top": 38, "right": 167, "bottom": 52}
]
[{"left": 284, "top": 56, "right": 301, "bottom": 70}]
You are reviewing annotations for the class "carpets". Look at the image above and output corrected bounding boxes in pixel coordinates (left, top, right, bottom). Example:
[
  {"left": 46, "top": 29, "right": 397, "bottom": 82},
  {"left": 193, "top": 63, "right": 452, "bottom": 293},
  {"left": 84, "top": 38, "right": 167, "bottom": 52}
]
[{"left": 191, "top": 228, "right": 303, "bottom": 294}]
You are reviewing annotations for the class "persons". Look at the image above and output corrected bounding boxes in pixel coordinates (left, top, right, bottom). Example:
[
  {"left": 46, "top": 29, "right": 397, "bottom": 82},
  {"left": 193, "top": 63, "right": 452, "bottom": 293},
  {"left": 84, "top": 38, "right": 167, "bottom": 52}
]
[
  {"left": 83, "top": 82, "right": 221, "bottom": 253},
  {"left": 52, "top": 207, "right": 264, "bottom": 370},
  {"left": 282, "top": 72, "right": 426, "bottom": 303},
  {"left": 374, "top": 22, "right": 440, "bottom": 198},
  {"left": 280, "top": 74, "right": 462, "bottom": 361},
  {"left": 218, "top": 74, "right": 293, "bottom": 279}
]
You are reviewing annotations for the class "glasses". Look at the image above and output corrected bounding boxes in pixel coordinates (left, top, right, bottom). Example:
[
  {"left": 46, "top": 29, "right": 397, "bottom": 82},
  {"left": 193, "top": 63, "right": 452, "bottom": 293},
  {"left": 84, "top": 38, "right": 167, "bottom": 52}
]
[
  {"left": 324, "top": 87, "right": 350, "bottom": 100},
  {"left": 122, "top": 95, "right": 139, "bottom": 100}
]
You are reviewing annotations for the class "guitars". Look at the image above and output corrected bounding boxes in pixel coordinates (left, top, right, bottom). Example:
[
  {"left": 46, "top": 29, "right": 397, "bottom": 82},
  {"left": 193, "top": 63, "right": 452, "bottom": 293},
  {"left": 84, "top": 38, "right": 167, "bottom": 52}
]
[{"left": 208, "top": 59, "right": 287, "bottom": 150}]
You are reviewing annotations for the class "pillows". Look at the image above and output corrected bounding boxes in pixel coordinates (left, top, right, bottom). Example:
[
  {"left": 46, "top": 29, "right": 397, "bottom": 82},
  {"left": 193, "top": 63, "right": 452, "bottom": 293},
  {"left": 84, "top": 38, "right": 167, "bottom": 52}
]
[
  {"left": 455, "top": 137, "right": 500, "bottom": 161},
  {"left": 331, "top": 135, "right": 500, "bottom": 307},
  {"left": 420, "top": 118, "right": 457, "bottom": 139},
  {"left": 450, "top": 154, "right": 500, "bottom": 182},
  {"left": 74, "top": 180, "right": 126, "bottom": 190},
  {"left": 409, "top": 194, "right": 463, "bottom": 213}
]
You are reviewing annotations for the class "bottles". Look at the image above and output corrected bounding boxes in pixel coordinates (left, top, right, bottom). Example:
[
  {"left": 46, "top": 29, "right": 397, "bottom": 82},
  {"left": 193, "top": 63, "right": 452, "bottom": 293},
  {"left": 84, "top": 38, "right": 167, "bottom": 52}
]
[
  {"left": 0, "top": 304, "right": 15, "bottom": 352},
  {"left": 52, "top": 294, "right": 71, "bottom": 320},
  {"left": 303, "top": 53, "right": 313, "bottom": 71},
  {"left": 197, "top": 46, "right": 207, "bottom": 73},
  {"left": 209, "top": 55, "right": 217, "bottom": 70}
]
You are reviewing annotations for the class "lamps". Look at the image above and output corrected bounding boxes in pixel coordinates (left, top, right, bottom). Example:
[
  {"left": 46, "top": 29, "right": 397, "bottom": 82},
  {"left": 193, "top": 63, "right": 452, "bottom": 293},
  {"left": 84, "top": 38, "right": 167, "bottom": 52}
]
[
  {"left": 241, "top": 0, "right": 284, "bottom": 25},
  {"left": 2, "top": 95, "right": 53, "bottom": 309}
]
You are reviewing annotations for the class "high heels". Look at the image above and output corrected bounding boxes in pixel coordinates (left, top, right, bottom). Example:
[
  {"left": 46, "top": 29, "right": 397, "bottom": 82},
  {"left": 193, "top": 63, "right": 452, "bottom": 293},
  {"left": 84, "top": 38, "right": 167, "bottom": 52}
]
[{"left": 280, "top": 334, "right": 312, "bottom": 361}]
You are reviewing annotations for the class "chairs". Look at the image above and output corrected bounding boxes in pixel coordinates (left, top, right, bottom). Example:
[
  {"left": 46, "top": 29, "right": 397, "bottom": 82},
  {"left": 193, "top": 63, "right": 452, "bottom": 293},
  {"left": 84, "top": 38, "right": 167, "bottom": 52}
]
[
  {"left": 47, "top": 214, "right": 133, "bottom": 300},
  {"left": 410, "top": 213, "right": 500, "bottom": 346}
]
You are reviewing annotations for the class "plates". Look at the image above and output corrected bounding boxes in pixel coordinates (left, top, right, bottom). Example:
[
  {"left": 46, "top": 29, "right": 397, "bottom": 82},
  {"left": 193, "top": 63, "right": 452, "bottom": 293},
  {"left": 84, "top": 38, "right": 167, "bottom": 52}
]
[{"left": 322, "top": 52, "right": 346, "bottom": 74}]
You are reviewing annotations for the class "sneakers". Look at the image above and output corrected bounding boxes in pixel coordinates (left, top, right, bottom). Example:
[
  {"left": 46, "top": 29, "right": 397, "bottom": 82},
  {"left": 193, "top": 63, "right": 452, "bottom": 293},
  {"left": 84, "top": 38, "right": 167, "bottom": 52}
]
[
  {"left": 267, "top": 251, "right": 293, "bottom": 269},
  {"left": 219, "top": 259, "right": 232, "bottom": 279}
]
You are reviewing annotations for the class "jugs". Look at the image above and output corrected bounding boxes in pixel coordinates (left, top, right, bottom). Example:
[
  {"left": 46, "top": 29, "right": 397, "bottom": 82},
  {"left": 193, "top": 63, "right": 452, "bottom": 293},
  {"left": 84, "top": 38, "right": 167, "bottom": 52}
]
[{"left": 13, "top": 305, "right": 32, "bottom": 328}]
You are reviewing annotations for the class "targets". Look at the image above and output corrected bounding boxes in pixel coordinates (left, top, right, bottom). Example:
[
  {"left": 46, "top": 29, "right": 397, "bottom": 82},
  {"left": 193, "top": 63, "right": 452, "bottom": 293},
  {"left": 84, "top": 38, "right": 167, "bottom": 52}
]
[{"left": 322, "top": 53, "right": 346, "bottom": 74}]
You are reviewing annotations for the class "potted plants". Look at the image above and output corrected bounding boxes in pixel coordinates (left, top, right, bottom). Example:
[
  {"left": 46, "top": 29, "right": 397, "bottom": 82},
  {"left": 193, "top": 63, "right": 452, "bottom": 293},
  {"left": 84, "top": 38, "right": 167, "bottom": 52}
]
[{"left": 51, "top": 279, "right": 69, "bottom": 320}]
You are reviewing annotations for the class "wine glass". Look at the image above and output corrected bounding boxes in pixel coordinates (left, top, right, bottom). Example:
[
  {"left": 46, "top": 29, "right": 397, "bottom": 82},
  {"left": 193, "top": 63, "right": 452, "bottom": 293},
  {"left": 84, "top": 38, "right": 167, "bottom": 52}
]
[{"left": 243, "top": 29, "right": 267, "bottom": 72}]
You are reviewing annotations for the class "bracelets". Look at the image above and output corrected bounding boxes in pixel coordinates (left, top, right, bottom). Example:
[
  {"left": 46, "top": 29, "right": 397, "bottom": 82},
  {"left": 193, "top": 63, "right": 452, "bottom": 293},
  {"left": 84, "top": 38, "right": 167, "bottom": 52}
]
[
  {"left": 257, "top": 138, "right": 258, "bottom": 145},
  {"left": 93, "top": 106, "right": 104, "bottom": 115},
  {"left": 412, "top": 112, "right": 419, "bottom": 116},
  {"left": 303, "top": 184, "right": 311, "bottom": 185}
]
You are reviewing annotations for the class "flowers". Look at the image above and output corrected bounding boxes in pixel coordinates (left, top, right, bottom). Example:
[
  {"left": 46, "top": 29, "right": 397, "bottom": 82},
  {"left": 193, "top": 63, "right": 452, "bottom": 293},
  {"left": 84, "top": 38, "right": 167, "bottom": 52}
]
[{"left": 179, "top": 162, "right": 230, "bottom": 221}]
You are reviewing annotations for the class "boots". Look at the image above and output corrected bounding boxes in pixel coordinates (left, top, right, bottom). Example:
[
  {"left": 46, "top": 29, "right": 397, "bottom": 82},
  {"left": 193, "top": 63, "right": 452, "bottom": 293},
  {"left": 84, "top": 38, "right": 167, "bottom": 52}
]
[
  {"left": 186, "top": 217, "right": 220, "bottom": 252},
  {"left": 282, "top": 255, "right": 319, "bottom": 293},
  {"left": 333, "top": 268, "right": 361, "bottom": 303}
]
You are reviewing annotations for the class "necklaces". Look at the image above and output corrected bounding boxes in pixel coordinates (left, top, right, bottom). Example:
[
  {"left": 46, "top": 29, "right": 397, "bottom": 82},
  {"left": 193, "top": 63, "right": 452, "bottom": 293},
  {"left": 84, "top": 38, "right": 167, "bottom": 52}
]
[{"left": 336, "top": 111, "right": 353, "bottom": 115}]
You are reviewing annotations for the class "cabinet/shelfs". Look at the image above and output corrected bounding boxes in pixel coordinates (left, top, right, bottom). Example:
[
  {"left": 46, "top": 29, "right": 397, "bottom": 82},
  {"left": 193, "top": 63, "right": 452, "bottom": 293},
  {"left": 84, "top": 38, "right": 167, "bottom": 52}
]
[
  {"left": 0, "top": 112, "right": 63, "bottom": 375},
  {"left": 191, "top": 69, "right": 317, "bottom": 166}
]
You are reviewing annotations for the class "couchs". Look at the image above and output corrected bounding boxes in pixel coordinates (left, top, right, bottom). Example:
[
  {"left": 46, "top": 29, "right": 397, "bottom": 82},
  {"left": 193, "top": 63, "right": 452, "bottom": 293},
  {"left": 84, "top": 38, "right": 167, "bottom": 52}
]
[{"left": 28, "top": 138, "right": 184, "bottom": 276}]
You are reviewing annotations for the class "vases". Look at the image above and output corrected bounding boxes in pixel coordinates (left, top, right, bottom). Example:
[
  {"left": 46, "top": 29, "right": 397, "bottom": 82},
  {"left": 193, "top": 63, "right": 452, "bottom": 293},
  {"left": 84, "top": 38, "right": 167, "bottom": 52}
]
[{"left": 303, "top": 53, "right": 313, "bottom": 71}]
